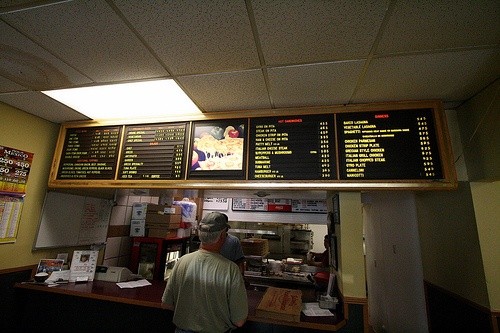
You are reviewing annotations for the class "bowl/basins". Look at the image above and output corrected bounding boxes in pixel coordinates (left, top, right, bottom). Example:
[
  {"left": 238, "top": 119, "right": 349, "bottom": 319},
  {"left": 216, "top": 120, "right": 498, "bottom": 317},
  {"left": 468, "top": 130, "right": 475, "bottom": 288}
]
[{"left": 34, "top": 274, "right": 48, "bottom": 282}]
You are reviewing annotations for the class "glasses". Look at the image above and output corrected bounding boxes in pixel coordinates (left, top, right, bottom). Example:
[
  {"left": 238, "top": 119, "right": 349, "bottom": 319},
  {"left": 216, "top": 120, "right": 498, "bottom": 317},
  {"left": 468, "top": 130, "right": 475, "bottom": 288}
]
[{"left": 221, "top": 231, "right": 228, "bottom": 238}]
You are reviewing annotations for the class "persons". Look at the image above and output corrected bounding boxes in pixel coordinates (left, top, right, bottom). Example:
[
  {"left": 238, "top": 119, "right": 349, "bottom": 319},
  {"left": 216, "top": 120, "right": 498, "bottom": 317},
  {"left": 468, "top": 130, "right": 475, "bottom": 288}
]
[
  {"left": 307, "top": 235, "right": 330, "bottom": 267},
  {"left": 161, "top": 213, "right": 248, "bottom": 333},
  {"left": 199, "top": 213, "right": 245, "bottom": 279}
]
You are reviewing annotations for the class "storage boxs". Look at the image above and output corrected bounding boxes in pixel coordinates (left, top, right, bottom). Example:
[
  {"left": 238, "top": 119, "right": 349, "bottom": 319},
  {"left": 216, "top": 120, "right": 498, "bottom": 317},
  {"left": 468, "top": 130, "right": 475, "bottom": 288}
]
[
  {"left": 241, "top": 238, "right": 268, "bottom": 256},
  {"left": 130, "top": 197, "right": 201, "bottom": 238},
  {"left": 255, "top": 287, "right": 303, "bottom": 322}
]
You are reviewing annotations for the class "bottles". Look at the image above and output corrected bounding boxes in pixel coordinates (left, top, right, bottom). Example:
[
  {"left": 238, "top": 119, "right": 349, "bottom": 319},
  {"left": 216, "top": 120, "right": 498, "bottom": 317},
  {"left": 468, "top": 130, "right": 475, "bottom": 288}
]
[
  {"left": 266, "top": 261, "right": 270, "bottom": 277},
  {"left": 244, "top": 262, "right": 246, "bottom": 271}
]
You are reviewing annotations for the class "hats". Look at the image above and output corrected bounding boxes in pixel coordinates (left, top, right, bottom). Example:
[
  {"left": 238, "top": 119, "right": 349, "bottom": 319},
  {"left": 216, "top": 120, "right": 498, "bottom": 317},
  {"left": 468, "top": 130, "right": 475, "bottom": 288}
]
[{"left": 197, "top": 212, "right": 231, "bottom": 233}]
